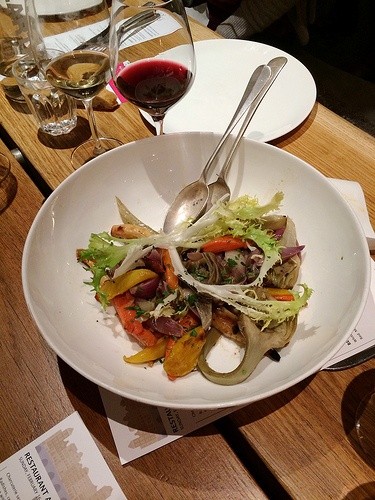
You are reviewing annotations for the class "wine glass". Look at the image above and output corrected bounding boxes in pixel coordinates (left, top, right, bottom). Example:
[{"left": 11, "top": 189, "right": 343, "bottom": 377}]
[
  {"left": 25, "top": 0, "right": 122, "bottom": 170},
  {"left": 109, "top": 0, "right": 196, "bottom": 137}
]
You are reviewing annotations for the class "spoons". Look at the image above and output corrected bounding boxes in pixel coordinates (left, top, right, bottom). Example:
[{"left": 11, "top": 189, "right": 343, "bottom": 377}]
[
  {"left": 162, "top": 54, "right": 287, "bottom": 231},
  {"left": 74, "top": 9, "right": 160, "bottom": 51}
]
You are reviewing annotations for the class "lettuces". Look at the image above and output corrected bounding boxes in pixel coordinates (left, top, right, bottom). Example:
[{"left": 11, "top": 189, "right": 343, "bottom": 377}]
[{"left": 77, "top": 192, "right": 313, "bottom": 325}]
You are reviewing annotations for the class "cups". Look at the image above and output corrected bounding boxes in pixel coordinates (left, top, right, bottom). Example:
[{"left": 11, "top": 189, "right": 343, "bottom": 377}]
[
  {"left": 12, "top": 49, "right": 77, "bottom": 136},
  {"left": 0, "top": 0, "right": 47, "bottom": 105},
  {"left": 0, "top": 151, "right": 11, "bottom": 213}
]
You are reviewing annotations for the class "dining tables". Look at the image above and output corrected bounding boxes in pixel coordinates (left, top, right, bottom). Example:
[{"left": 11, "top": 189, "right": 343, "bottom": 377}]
[{"left": 0, "top": 0, "right": 375, "bottom": 500}]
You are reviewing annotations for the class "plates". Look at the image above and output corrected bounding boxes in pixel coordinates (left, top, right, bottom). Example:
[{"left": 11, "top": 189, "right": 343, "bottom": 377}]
[
  {"left": 21, "top": 132, "right": 370, "bottom": 409},
  {"left": 137, "top": 39, "right": 316, "bottom": 142},
  {"left": 0, "top": 0, "right": 103, "bottom": 16}
]
